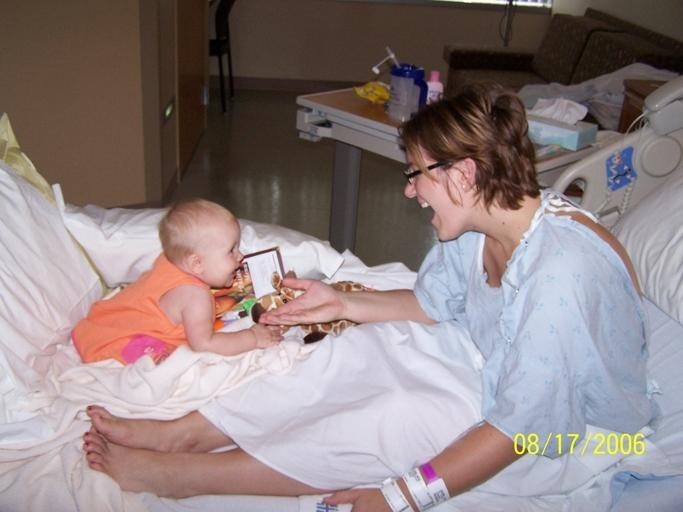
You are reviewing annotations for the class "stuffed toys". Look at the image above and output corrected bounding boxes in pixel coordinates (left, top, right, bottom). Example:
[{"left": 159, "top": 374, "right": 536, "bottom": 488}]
[{"left": 252, "top": 271, "right": 380, "bottom": 344}]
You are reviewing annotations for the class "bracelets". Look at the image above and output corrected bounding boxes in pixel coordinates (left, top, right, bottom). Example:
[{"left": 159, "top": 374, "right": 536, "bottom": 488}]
[
  {"left": 400, "top": 468, "right": 433, "bottom": 512},
  {"left": 378, "top": 477, "right": 415, "bottom": 512},
  {"left": 420, "top": 462, "right": 449, "bottom": 506}
]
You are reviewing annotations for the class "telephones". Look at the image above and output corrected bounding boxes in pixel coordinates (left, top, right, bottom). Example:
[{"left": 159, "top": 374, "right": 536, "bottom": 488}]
[{"left": 642, "top": 75, "right": 683, "bottom": 137}]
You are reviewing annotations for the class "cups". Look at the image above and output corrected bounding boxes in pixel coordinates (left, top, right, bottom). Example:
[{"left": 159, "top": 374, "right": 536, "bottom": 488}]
[{"left": 387, "top": 63, "right": 429, "bottom": 124}]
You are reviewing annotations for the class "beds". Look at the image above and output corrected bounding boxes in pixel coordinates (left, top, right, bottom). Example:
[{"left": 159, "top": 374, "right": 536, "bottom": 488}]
[{"left": 0, "top": 116, "right": 683, "bottom": 512}]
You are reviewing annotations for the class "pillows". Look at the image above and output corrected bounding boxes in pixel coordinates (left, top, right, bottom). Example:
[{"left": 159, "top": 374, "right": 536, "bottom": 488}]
[
  {"left": 609, "top": 162, "right": 683, "bottom": 324},
  {"left": 1, "top": 113, "right": 106, "bottom": 404},
  {"left": 532, "top": 13, "right": 623, "bottom": 85},
  {"left": 56, "top": 181, "right": 342, "bottom": 289},
  {"left": 570, "top": 30, "right": 672, "bottom": 86}
]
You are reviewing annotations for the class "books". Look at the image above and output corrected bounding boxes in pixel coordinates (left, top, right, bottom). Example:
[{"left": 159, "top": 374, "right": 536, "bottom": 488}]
[{"left": 207, "top": 245, "right": 286, "bottom": 335}]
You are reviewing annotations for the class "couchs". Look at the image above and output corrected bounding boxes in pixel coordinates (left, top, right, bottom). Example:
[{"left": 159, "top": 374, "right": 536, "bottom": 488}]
[{"left": 441, "top": 7, "right": 683, "bottom": 97}]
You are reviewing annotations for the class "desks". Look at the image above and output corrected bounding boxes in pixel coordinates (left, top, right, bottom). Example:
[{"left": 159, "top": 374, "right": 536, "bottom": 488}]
[{"left": 295, "top": 80, "right": 593, "bottom": 257}]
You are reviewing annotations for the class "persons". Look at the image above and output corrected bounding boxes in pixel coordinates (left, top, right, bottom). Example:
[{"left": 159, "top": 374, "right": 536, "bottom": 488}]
[
  {"left": 67, "top": 196, "right": 284, "bottom": 369},
  {"left": 84, "top": 80, "right": 653, "bottom": 512}
]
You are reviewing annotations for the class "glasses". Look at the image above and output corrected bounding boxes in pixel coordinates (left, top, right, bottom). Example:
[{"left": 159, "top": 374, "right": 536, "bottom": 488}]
[{"left": 401, "top": 159, "right": 447, "bottom": 184}]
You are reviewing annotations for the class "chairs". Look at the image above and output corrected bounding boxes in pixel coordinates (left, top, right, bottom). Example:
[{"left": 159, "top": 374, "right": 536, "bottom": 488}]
[{"left": 209, "top": 0, "right": 235, "bottom": 111}]
